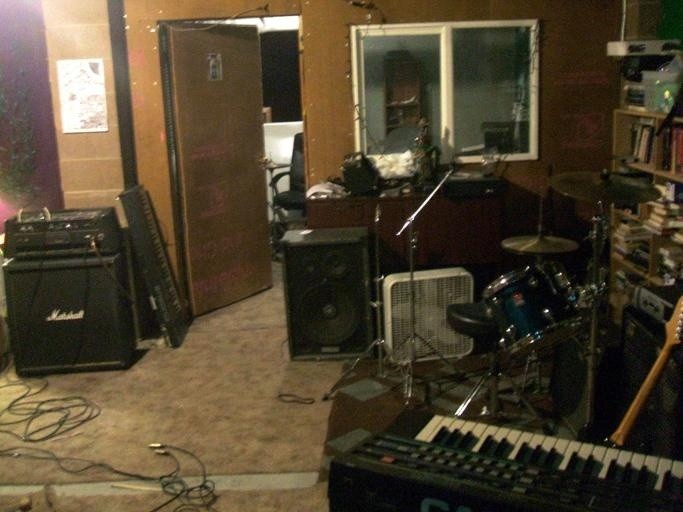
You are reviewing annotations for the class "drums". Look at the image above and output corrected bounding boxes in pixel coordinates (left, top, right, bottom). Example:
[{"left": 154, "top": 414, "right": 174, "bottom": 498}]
[
  {"left": 540, "top": 261, "right": 593, "bottom": 329},
  {"left": 483, "top": 265, "right": 586, "bottom": 367}
]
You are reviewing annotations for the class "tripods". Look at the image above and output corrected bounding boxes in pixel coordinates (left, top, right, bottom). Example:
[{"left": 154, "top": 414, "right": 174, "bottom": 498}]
[
  {"left": 319, "top": 235, "right": 401, "bottom": 402},
  {"left": 383, "top": 184, "right": 465, "bottom": 415}
]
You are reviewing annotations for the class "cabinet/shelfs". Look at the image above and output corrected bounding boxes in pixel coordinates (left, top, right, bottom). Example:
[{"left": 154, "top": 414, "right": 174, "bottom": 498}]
[
  {"left": 605, "top": 107, "right": 680, "bottom": 458},
  {"left": 384, "top": 59, "right": 422, "bottom": 140}
]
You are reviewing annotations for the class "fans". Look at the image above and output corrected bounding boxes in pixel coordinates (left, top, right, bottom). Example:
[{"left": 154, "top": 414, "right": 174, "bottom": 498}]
[{"left": 382, "top": 268, "right": 475, "bottom": 366}]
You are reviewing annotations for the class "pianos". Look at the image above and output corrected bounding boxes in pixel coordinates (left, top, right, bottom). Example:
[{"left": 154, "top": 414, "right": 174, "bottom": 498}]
[
  {"left": 327, "top": 402, "right": 683, "bottom": 512},
  {"left": 118, "top": 183, "right": 191, "bottom": 348}
]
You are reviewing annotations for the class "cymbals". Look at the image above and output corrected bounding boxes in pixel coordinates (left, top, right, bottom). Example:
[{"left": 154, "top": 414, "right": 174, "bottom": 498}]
[
  {"left": 499, "top": 234, "right": 579, "bottom": 254},
  {"left": 548, "top": 169, "right": 662, "bottom": 205}
]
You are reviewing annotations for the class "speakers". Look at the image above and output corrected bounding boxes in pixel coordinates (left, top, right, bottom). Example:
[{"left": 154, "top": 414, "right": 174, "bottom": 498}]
[
  {"left": 280, "top": 227, "right": 373, "bottom": 364},
  {"left": 1, "top": 253, "right": 140, "bottom": 376}
]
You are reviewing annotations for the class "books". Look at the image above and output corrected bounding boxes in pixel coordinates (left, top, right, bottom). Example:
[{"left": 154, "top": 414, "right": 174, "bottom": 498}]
[{"left": 606, "top": 118, "right": 682, "bottom": 304}]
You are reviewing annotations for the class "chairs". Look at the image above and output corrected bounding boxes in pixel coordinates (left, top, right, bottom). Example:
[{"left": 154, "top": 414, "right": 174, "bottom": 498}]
[{"left": 268, "top": 133, "right": 306, "bottom": 262}]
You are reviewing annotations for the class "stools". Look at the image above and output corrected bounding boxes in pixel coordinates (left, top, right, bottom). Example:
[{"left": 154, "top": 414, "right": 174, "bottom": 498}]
[{"left": 447, "top": 302, "right": 558, "bottom": 434}]
